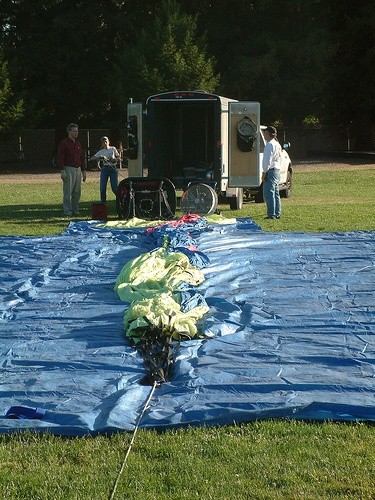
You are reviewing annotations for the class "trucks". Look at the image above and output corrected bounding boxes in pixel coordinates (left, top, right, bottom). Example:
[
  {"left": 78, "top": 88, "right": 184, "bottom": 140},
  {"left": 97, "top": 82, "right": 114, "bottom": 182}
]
[{"left": 125, "top": 89, "right": 294, "bottom": 212}]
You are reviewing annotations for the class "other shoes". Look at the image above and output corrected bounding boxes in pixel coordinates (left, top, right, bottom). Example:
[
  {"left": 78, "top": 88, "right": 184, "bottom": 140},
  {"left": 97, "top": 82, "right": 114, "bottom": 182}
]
[{"left": 264, "top": 216, "right": 281, "bottom": 219}]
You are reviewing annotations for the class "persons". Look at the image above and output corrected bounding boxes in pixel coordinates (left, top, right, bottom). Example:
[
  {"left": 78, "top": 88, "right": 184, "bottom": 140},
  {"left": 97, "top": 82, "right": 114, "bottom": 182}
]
[
  {"left": 58, "top": 123, "right": 86, "bottom": 215},
  {"left": 261, "top": 126, "right": 281, "bottom": 219},
  {"left": 91, "top": 136, "right": 120, "bottom": 204}
]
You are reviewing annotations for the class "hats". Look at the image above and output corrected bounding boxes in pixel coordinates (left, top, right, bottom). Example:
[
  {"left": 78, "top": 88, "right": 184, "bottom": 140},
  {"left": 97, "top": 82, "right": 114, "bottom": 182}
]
[{"left": 261, "top": 126, "right": 277, "bottom": 136}]
